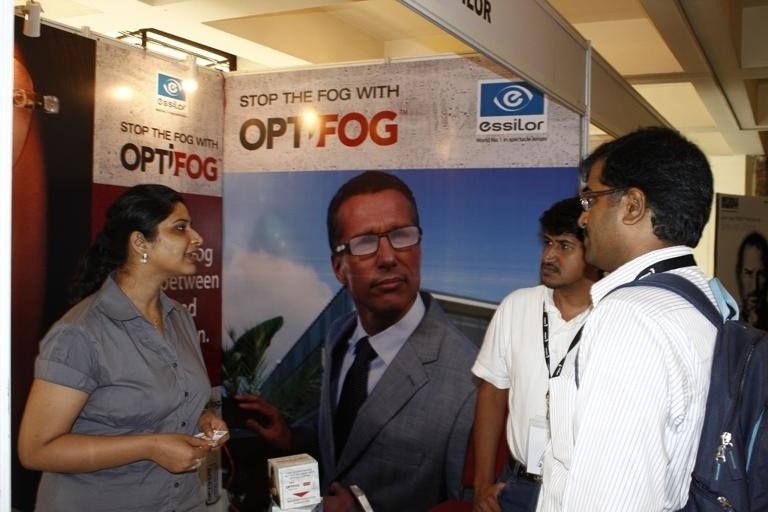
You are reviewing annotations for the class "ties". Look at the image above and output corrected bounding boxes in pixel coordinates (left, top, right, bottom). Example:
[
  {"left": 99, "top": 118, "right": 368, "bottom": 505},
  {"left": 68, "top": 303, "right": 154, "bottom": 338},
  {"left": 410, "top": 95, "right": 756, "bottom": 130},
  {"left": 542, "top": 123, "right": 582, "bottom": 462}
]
[{"left": 332, "top": 339, "right": 377, "bottom": 461}]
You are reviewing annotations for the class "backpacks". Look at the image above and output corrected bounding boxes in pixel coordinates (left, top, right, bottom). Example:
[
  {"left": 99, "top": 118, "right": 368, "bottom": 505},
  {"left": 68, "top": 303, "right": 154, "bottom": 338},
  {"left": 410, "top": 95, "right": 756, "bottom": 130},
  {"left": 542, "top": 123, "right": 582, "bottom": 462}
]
[{"left": 574, "top": 273, "right": 768, "bottom": 511}]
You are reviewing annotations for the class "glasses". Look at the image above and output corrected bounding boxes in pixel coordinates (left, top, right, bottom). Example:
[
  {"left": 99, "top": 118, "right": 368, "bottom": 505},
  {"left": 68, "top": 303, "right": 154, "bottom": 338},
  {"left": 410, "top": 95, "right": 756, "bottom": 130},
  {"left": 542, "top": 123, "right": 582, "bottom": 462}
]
[
  {"left": 579, "top": 187, "right": 623, "bottom": 212},
  {"left": 335, "top": 224, "right": 422, "bottom": 256}
]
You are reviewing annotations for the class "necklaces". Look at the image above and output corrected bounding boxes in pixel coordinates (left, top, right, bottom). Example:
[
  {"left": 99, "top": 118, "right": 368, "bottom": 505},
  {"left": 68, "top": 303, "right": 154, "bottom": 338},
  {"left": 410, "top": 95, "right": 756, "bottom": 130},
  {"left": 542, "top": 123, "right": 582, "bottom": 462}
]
[{"left": 149, "top": 315, "right": 159, "bottom": 329}]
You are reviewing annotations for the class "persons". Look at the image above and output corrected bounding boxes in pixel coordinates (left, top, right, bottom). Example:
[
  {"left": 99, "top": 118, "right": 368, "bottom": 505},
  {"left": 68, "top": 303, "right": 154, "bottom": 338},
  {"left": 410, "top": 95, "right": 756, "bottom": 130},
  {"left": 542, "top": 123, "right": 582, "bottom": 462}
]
[
  {"left": 234, "top": 169, "right": 482, "bottom": 512},
  {"left": 734, "top": 230, "right": 767, "bottom": 331},
  {"left": 470, "top": 194, "right": 606, "bottom": 512},
  {"left": 16, "top": 182, "right": 234, "bottom": 512},
  {"left": 532, "top": 122, "right": 768, "bottom": 512}
]
